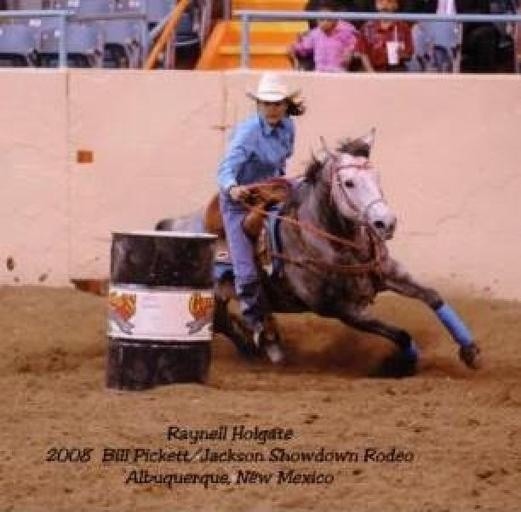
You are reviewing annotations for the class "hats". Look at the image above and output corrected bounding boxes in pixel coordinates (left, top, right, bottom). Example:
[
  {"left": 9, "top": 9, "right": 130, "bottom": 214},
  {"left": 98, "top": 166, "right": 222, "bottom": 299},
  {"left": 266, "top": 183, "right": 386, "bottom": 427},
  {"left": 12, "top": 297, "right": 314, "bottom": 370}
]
[{"left": 244, "top": 71, "right": 303, "bottom": 103}]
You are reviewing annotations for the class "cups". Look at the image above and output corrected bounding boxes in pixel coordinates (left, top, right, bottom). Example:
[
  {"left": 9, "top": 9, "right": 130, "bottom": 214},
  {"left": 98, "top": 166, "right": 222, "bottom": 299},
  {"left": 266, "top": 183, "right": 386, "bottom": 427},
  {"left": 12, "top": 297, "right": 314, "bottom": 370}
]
[{"left": 386, "top": 40, "right": 399, "bottom": 64}]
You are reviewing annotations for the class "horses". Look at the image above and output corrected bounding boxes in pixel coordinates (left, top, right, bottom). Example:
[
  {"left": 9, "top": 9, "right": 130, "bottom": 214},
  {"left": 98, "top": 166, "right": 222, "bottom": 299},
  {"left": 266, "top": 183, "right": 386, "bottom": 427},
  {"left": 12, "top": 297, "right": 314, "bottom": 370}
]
[{"left": 68, "top": 127, "right": 484, "bottom": 379}]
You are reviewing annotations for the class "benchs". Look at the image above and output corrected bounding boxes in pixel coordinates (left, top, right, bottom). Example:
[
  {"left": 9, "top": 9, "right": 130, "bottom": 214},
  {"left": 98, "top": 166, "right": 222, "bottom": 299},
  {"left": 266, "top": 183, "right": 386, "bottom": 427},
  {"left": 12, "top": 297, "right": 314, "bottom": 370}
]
[{"left": 0, "top": 0, "right": 521, "bottom": 67}]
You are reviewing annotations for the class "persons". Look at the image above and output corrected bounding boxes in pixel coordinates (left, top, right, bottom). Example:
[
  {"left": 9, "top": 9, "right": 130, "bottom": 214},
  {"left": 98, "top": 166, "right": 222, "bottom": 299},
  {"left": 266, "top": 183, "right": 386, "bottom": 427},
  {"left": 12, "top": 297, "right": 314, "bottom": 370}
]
[
  {"left": 353, "top": 0, "right": 414, "bottom": 71},
  {"left": 214, "top": 73, "right": 310, "bottom": 366},
  {"left": 290, "top": 4, "right": 358, "bottom": 71}
]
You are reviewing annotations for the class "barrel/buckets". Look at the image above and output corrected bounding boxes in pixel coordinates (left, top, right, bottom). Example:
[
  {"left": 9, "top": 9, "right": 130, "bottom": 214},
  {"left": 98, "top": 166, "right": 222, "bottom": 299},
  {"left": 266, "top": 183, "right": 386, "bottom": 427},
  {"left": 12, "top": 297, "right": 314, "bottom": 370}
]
[{"left": 107, "top": 231, "right": 218, "bottom": 388}]
